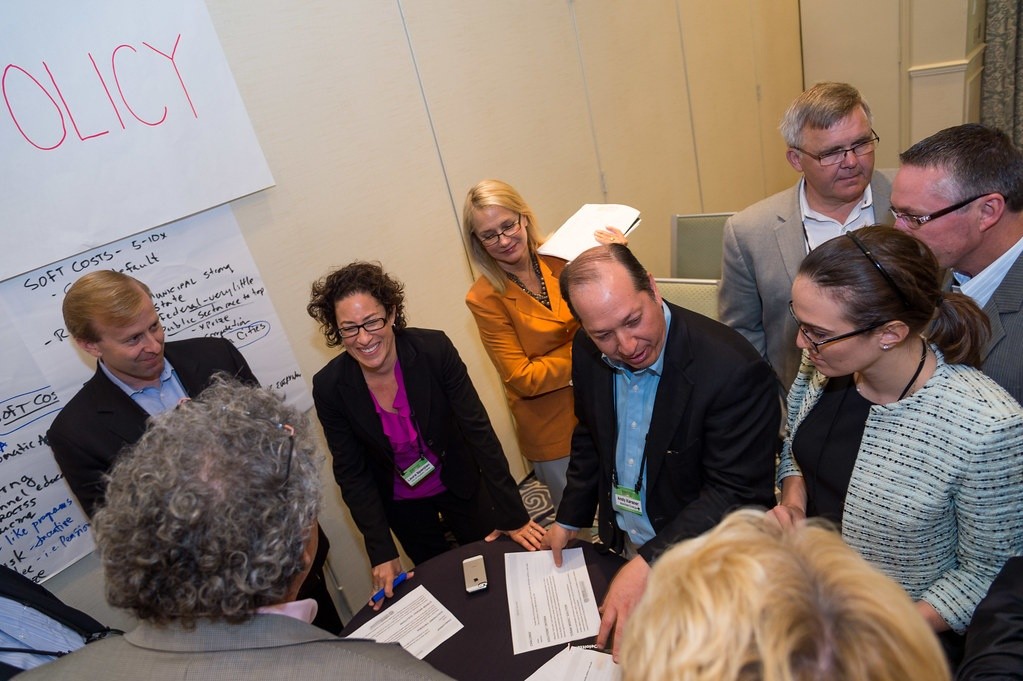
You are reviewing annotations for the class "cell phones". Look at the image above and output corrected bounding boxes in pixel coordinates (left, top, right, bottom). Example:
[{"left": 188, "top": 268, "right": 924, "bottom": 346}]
[{"left": 462, "top": 555, "right": 487, "bottom": 593}]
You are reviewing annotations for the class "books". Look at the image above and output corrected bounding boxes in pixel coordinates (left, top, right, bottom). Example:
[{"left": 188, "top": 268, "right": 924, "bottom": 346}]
[{"left": 535, "top": 202, "right": 642, "bottom": 263}]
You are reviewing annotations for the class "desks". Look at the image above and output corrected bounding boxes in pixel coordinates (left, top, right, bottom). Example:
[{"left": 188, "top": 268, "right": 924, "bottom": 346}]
[{"left": 339, "top": 535, "right": 633, "bottom": 681}]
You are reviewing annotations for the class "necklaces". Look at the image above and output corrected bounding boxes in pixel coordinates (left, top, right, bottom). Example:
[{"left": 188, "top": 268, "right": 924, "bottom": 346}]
[{"left": 855, "top": 333, "right": 927, "bottom": 404}]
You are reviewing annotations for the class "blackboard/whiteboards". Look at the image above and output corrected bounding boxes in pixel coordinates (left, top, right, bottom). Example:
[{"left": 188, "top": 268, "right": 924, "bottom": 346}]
[{"left": 0, "top": 2, "right": 318, "bottom": 585}]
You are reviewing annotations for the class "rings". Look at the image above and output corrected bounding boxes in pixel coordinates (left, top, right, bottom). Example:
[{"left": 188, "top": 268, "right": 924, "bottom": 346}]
[
  {"left": 610, "top": 236, "right": 615, "bottom": 240},
  {"left": 529, "top": 527, "right": 534, "bottom": 533}
]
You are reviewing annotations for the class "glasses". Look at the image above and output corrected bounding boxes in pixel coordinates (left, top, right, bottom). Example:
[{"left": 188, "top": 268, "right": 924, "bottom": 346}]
[
  {"left": 174, "top": 397, "right": 293, "bottom": 500},
  {"left": 788, "top": 300, "right": 913, "bottom": 354},
  {"left": 790, "top": 127, "right": 880, "bottom": 166},
  {"left": 335, "top": 309, "right": 388, "bottom": 338},
  {"left": 473, "top": 213, "right": 522, "bottom": 248},
  {"left": 888, "top": 192, "right": 1009, "bottom": 230}
]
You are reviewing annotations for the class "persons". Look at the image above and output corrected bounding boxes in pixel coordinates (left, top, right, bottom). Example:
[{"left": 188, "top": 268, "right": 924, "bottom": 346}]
[
  {"left": 307, "top": 259, "right": 548, "bottom": 612},
  {"left": 538, "top": 243, "right": 781, "bottom": 666},
  {"left": 462, "top": 178, "right": 630, "bottom": 513},
  {"left": 45, "top": 269, "right": 345, "bottom": 635},
  {"left": 0, "top": 561, "right": 126, "bottom": 681},
  {"left": 614, "top": 78, "right": 1023, "bottom": 681},
  {"left": 14, "top": 367, "right": 462, "bottom": 681}
]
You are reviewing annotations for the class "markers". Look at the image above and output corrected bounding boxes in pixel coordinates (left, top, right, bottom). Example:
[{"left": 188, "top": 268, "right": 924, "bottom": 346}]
[{"left": 371, "top": 572, "right": 408, "bottom": 604}]
